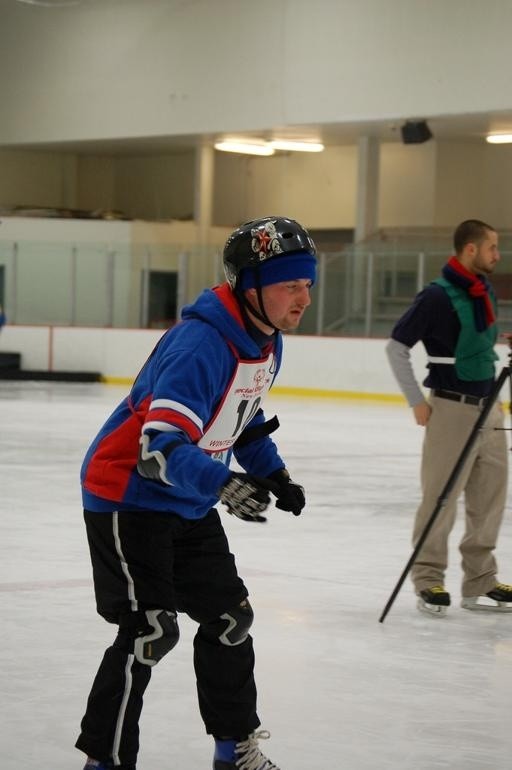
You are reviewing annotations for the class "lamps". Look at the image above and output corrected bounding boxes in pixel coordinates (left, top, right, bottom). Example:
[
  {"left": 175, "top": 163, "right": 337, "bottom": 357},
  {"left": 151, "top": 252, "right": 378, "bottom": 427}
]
[{"left": 400, "top": 119, "right": 433, "bottom": 144}]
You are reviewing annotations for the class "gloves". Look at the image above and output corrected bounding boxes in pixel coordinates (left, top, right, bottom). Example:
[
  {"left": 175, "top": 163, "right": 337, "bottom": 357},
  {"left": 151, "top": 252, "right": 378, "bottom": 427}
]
[
  {"left": 217, "top": 469, "right": 270, "bottom": 525},
  {"left": 263, "top": 466, "right": 308, "bottom": 517}
]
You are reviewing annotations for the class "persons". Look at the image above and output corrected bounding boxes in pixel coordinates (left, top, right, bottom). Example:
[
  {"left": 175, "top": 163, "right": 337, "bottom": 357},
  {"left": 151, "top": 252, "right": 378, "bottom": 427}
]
[
  {"left": 72, "top": 213, "right": 310, "bottom": 768},
  {"left": 379, "top": 212, "right": 512, "bottom": 615}
]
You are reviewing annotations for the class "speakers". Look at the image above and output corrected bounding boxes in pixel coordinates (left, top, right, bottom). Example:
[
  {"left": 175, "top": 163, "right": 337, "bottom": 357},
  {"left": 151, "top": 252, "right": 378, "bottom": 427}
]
[{"left": 402, "top": 121, "right": 431, "bottom": 143}]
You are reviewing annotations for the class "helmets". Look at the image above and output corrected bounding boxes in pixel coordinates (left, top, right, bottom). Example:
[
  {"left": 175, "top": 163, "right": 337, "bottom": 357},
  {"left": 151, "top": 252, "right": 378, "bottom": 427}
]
[{"left": 220, "top": 213, "right": 319, "bottom": 294}]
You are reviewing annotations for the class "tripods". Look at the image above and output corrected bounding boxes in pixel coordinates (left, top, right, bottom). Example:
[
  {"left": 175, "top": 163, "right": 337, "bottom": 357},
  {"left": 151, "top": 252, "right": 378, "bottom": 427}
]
[{"left": 379, "top": 335, "right": 512, "bottom": 623}]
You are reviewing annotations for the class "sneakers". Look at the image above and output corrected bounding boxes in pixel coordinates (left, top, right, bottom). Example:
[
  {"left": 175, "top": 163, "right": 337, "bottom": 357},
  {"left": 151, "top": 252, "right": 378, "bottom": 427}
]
[
  {"left": 479, "top": 583, "right": 512, "bottom": 604},
  {"left": 209, "top": 730, "right": 285, "bottom": 770},
  {"left": 418, "top": 585, "right": 452, "bottom": 608}
]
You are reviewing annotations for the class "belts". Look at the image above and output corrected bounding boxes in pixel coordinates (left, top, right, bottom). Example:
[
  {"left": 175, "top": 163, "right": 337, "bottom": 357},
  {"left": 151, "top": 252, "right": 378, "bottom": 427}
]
[{"left": 429, "top": 387, "right": 490, "bottom": 408}]
